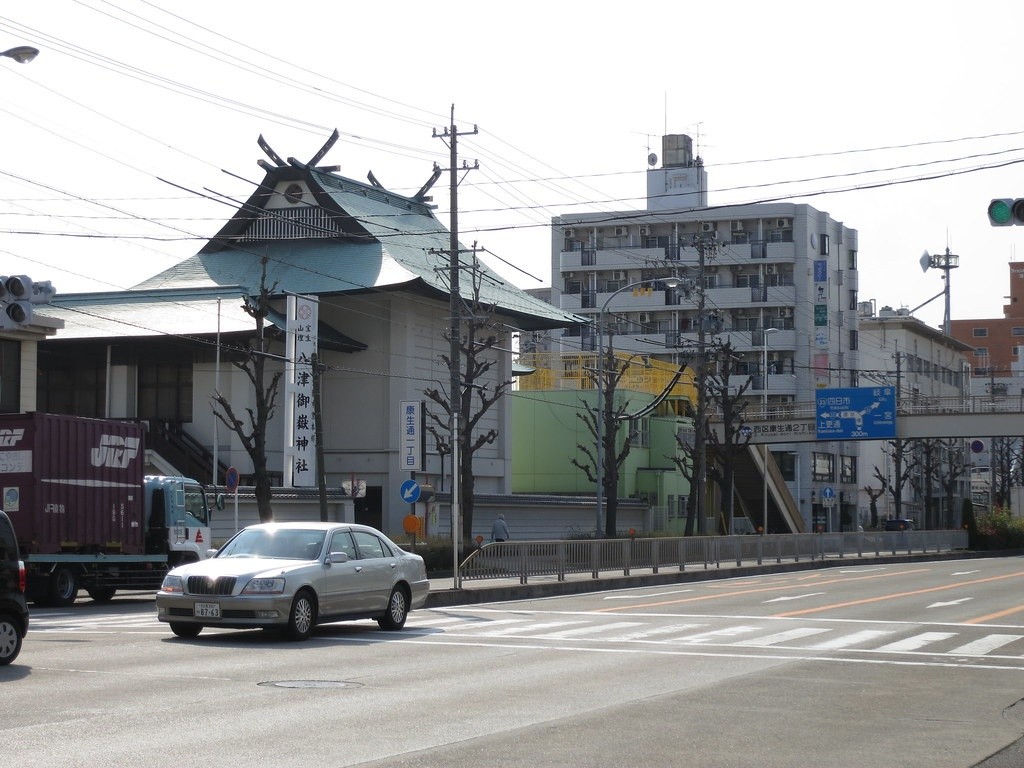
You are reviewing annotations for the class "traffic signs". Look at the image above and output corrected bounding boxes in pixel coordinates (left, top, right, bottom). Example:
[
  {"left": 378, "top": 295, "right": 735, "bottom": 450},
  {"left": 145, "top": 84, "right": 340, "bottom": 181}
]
[{"left": 815, "top": 383, "right": 899, "bottom": 441}]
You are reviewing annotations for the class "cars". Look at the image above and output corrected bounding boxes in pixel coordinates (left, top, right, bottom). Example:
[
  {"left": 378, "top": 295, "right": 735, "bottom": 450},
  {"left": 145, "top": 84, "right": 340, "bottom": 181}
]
[
  {"left": 0, "top": 510, "right": 30, "bottom": 665},
  {"left": 154, "top": 522, "right": 431, "bottom": 642}
]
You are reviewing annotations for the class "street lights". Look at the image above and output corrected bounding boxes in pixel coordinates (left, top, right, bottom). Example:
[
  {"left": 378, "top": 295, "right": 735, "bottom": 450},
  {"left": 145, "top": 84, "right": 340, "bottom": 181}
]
[
  {"left": 760, "top": 327, "right": 781, "bottom": 537},
  {"left": 593, "top": 274, "right": 682, "bottom": 533}
]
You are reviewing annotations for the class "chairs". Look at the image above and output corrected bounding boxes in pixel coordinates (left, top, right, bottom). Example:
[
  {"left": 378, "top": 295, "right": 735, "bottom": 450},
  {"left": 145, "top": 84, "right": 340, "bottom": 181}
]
[{"left": 311, "top": 541, "right": 343, "bottom": 557}]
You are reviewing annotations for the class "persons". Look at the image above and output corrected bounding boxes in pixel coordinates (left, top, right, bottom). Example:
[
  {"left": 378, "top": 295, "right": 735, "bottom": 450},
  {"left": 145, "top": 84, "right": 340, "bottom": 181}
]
[{"left": 490, "top": 513, "right": 510, "bottom": 541}]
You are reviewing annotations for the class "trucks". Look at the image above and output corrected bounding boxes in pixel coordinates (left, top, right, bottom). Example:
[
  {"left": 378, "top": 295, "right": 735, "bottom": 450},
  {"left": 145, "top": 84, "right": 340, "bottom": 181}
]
[{"left": 0, "top": 409, "right": 227, "bottom": 609}]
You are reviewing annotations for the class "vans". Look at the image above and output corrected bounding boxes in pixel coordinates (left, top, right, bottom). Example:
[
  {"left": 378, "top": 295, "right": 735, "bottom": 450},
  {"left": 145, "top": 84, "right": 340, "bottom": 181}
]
[{"left": 885, "top": 518, "right": 917, "bottom": 530}]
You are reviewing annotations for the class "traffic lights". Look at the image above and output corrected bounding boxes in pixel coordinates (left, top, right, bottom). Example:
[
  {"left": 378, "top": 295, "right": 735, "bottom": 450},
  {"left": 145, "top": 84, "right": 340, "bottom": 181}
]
[{"left": 987, "top": 198, "right": 1024, "bottom": 226}]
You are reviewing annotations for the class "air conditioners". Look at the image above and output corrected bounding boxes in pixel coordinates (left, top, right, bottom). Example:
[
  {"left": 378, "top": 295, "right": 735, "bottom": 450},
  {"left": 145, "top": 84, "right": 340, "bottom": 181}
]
[
  {"left": 729, "top": 266, "right": 736, "bottom": 272},
  {"left": 613, "top": 271, "right": 625, "bottom": 281},
  {"left": 729, "top": 222, "right": 744, "bottom": 232},
  {"left": 638, "top": 313, "right": 650, "bottom": 323},
  {"left": 561, "top": 272, "right": 571, "bottom": 279},
  {"left": 615, "top": 227, "right": 628, "bottom": 237},
  {"left": 780, "top": 396, "right": 793, "bottom": 406},
  {"left": 778, "top": 309, "right": 791, "bottom": 317},
  {"left": 614, "top": 315, "right": 628, "bottom": 324},
  {"left": 639, "top": 227, "right": 652, "bottom": 236},
  {"left": 642, "top": 269, "right": 654, "bottom": 277},
  {"left": 705, "top": 354, "right": 716, "bottom": 363},
  {"left": 702, "top": 222, "right": 715, "bottom": 232},
  {"left": 776, "top": 218, "right": 790, "bottom": 229},
  {"left": 768, "top": 352, "right": 780, "bottom": 362},
  {"left": 564, "top": 228, "right": 575, "bottom": 239},
  {"left": 705, "top": 266, "right": 716, "bottom": 273}
]
